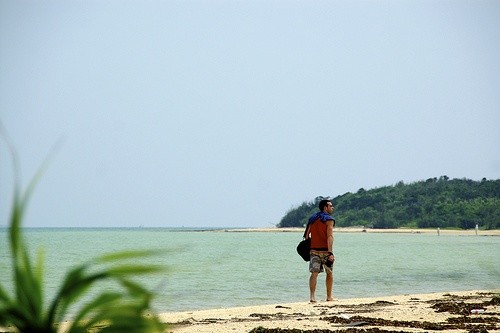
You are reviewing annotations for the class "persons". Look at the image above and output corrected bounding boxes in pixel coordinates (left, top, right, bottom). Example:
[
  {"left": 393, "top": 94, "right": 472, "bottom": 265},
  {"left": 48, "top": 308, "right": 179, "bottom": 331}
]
[{"left": 304, "top": 199, "right": 339, "bottom": 302}]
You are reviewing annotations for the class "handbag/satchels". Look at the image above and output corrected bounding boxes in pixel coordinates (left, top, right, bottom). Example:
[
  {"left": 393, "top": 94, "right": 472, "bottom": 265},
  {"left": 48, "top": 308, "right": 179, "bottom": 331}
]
[{"left": 296, "top": 237, "right": 312, "bottom": 262}]
